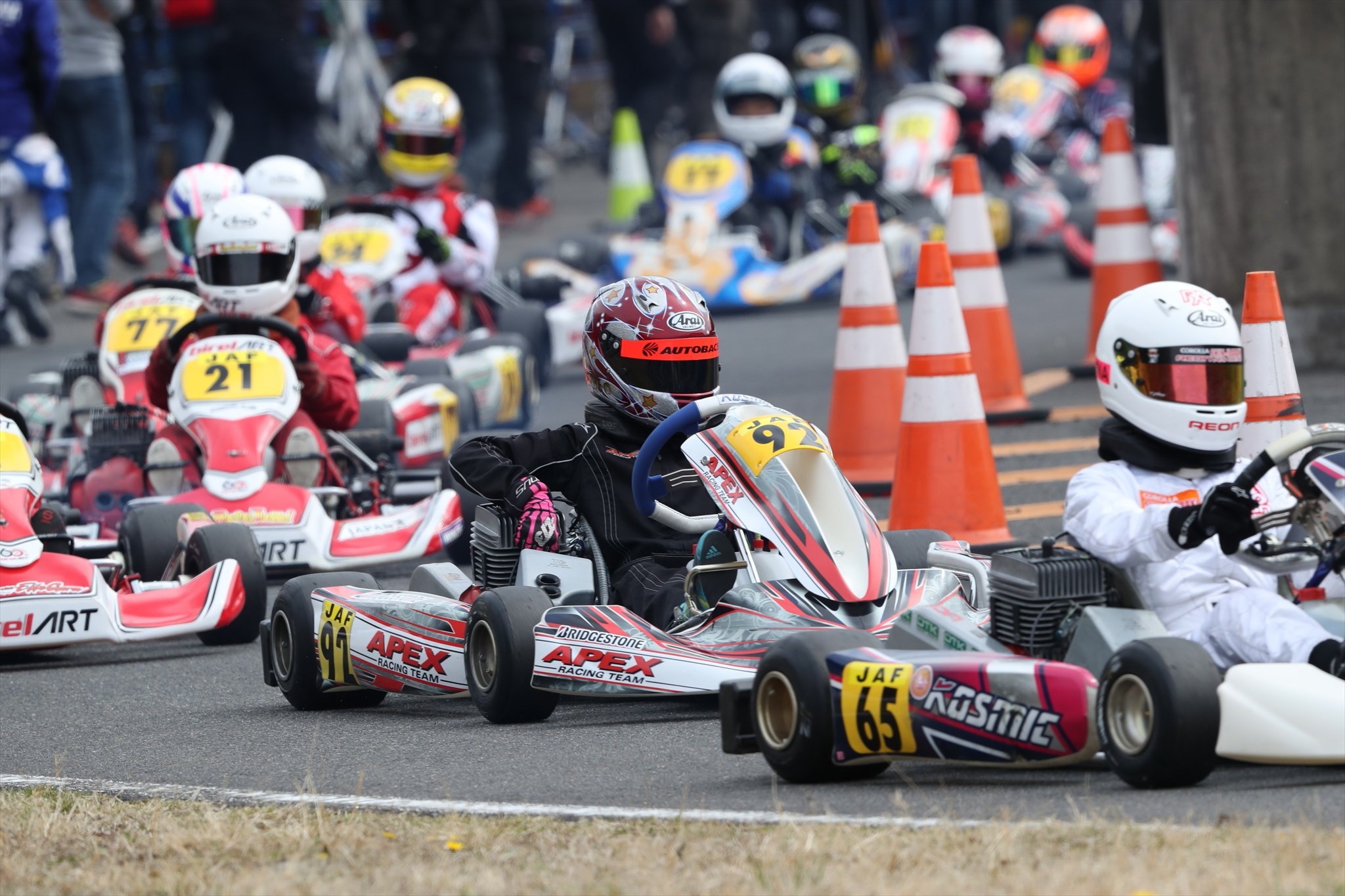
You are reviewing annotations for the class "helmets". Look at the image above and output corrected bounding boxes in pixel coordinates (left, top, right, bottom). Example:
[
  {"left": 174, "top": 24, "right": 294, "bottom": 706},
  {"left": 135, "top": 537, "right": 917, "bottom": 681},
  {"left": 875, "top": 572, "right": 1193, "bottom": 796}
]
[
  {"left": 187, "top": 193, "right": 300, "bottom": 316},
  {"left": 792, "top": 33, "right": 863, "bottom": 115},
  {"left": 1029, "top": 5, "right": 1110, "bottom": 91},
  {"left": 377, "top": 77, "right": 464, "bottom": 188},
  {"left": 715, "top": 54, "right": 797, "bottom": 145},
  {"left": 245, "top": 155, "right": 325, "bottom": 263},
  {"left": 582, "top": 275, "right": 721, "bottom": 425},
  {"left": 164, "top": 163, "right": 242, "bottom": 281},
  {"left": 1093, "top": 281, "right": 1247, "bottom": 452},
  {"left": 931, "top": 23, "right": 1006, "bottom": 110}
]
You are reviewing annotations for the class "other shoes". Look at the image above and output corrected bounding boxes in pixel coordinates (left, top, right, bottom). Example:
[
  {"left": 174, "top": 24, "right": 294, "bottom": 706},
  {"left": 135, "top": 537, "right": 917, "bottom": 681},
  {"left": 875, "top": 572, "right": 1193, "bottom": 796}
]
[
  {"left": 674, "top": 530, "right": 740, "bottom": 616},
  {"left": 497, "top": 195, "right": 553, "bottom": 229},
  {"left": 70, "top": 281, "right": 126, "bottom": 316},
  {"left": 114, "top": 221, "right": 150, "bottom": 267}
]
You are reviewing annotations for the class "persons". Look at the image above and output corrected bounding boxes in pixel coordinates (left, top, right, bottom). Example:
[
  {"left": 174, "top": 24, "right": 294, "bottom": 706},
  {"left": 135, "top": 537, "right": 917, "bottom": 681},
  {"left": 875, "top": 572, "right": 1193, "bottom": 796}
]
[
  {"left": 0, "top": 401, "right": 70, "bottom": 554},
  {"left": 146, "top": 194, "right": 360, "bottom": 496},
  {"left": 446, "top": 276, "right": 739, "bottom": 633},
  {"left": 1062, "top": 280, "right": 1345, "bottom": 681},
  {"left": 593, "top": 0, "right": 1137, "bottom": 261},
  {"left": 0, "top": 0, "right": 620, "bottom": 354}
]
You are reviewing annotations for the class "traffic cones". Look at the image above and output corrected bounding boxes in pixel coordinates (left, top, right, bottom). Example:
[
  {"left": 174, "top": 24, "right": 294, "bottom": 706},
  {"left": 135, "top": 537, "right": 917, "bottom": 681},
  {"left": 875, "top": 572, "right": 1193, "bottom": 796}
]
[
  {"left": 876, "top": 239, "right": 1031, "bottom": 559},
  {"left": 1061, "top": 112, "right": 1165, "bottom": 378},
  {"left": 936, "top": 150, "right": 1060, "bottom": 425},
  {"left": 814, "top": 200, "right": 914, "bottom": 497},
  {"left": 1241, "top": 271, "right": 1313, "bottom": 469},
  {"left": 592, "top": 105, "right": 659, "bottom": 239}
]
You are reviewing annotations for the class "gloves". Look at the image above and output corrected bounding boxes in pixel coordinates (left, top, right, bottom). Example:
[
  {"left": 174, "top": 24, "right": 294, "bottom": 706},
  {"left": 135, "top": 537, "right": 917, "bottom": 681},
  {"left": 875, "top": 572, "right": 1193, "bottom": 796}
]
[
  {"left": 296, "top": 359, "right": 323, "bottom": 393},
  {"left": 150, "top": 337, "right": 179, "bottom": 380},
  {"left": 508, "top": 474, "right": 561, "bottom": 553},
  {"left": 758, "top": 170, "right": 791, "bottom": 205},
  {"left": 415, "top": 228, "right": 449, "bottom": 264},
  {"left": 295, "top": 281, "right": 323, "bottom": 313},
  {"left": 987, "top": 136, "right": 1013, "bottom": 174},
  {"left": 1169, "top": 483, "right": 1259, "bottom": 551}
]
